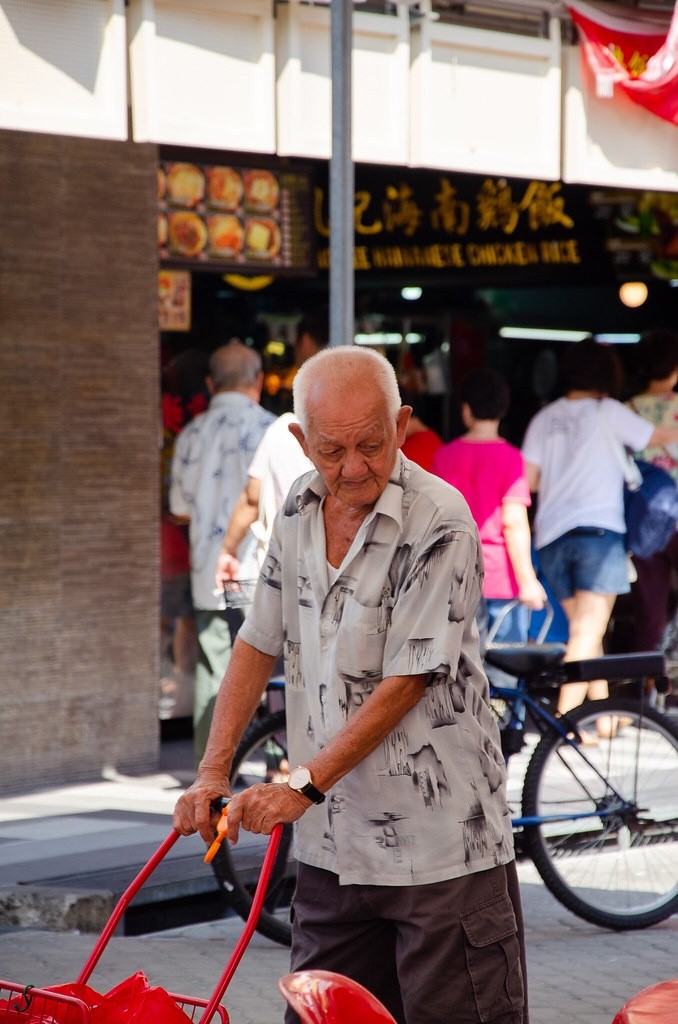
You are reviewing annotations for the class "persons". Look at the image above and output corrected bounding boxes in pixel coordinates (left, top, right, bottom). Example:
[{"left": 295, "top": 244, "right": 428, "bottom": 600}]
[
  {"left": 168, "top": 344, "right": 288, "bottom": 787},
  {"left": 520, "top": 347, "right": 678, "bottom": 744},
  {"left": 295, "top": 310, "right": 329, "bottom": 367},
  {"left": 613, "top": 335, "right": 678, "bottom": 703},
  {"left": 434, "top": 374, "right": 547, "bottom": 687},
  {"left": 213, "top": 412, "right": 316, "bottom": 744},
  {"left": 172, "top": 344, "right": 530, "bottom": 1024},
  {"left": 400, "top": 400, "right": 444, "bottom": 470}
]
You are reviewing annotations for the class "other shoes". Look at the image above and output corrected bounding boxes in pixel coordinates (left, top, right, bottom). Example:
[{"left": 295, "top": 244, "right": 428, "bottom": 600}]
[
  {"left": 596, "top": 713, "right": 633, "bottom": 739},
  {"left": 567, "top": 730, "right": 601, "bottom": 747}
]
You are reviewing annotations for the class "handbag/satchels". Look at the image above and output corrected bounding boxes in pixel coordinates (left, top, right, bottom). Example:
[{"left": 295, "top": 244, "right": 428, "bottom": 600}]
[{"left": 620, "top": 452, "right": 677, "bottom": 559}]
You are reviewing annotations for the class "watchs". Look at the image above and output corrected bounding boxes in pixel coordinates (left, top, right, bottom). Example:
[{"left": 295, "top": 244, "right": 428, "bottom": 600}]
[{"left": 288, "top": 766, "right": 326, "bottom": 805}]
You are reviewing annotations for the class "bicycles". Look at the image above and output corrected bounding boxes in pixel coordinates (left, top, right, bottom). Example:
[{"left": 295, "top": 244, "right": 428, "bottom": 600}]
[{"left": 207, "top": 642, "right": 677, "bottom": 943}]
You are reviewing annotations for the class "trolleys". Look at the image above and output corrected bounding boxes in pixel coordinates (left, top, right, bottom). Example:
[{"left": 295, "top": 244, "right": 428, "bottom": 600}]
[{"left": 1, "top": 797, "right": 286, "bottom": 1024}]
